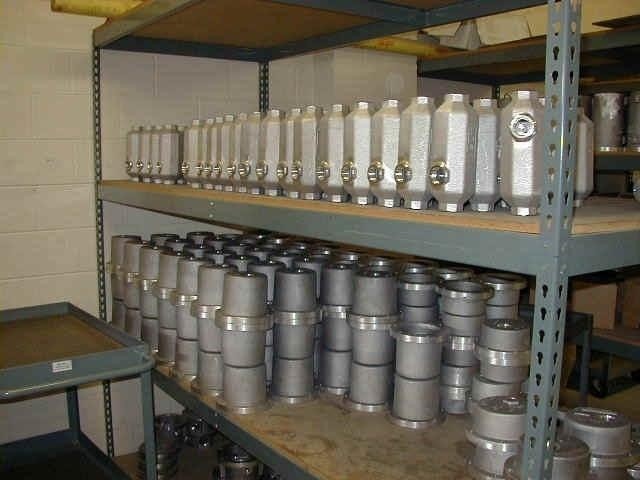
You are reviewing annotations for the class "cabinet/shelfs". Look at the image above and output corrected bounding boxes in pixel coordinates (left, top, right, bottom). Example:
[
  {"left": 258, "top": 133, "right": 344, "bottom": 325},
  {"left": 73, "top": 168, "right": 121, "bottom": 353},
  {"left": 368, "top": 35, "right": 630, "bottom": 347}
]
[{"left": 2, "top": 301, "right": 159, "bottom": 480}]
